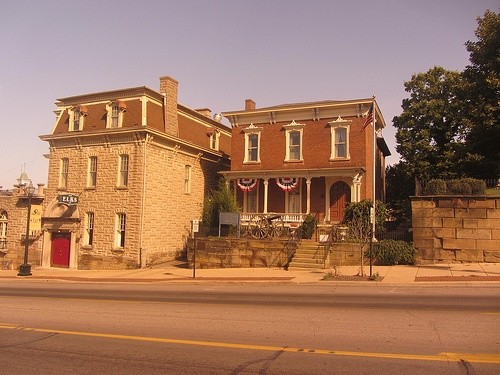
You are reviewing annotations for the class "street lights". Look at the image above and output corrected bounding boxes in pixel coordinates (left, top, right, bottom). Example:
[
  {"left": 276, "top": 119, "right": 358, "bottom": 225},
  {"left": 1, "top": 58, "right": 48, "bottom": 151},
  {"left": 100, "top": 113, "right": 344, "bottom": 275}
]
[{"left": 17, "top": 181, "right": 36, "bottom": 276}]
